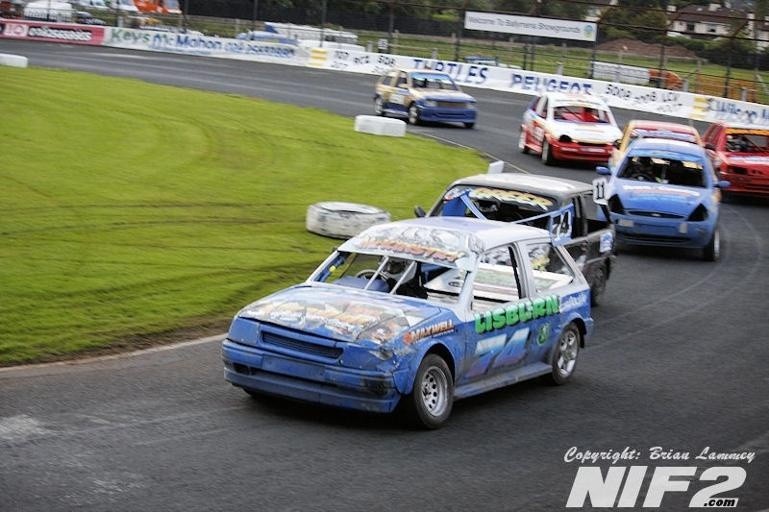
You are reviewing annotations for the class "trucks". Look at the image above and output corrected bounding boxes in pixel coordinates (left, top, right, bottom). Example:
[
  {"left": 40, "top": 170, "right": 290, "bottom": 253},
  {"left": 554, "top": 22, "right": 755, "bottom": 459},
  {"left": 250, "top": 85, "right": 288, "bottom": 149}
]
[
  {"left": 82, "top": 1, "right": 182, "bottom": 14},
  {"left": 591, "top": 61, "right": 683, "bottom": 89}
]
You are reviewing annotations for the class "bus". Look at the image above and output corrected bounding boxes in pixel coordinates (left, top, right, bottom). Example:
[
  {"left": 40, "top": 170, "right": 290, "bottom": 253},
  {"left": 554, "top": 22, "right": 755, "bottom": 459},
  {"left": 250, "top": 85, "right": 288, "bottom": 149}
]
[{"left": 265, "top": 22, "right": 357, "bottom": 44}]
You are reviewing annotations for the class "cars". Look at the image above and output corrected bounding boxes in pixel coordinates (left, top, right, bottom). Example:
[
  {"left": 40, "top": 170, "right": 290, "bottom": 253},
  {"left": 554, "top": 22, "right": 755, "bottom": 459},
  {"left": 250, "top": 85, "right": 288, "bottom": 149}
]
[
  {"left": 518, "top": 91, "right": 624, "bottom": 165},
  {"left": 375, "top": 67, "right": 476, "bottom": 129},
  {"left": 595, "top": 119, "right": 732, "bottom": 260},
  {"left": 703, "top": 121, "right": 769, "bottom": 201},
  {"left": 221, "top": 215, "right": 592, "bottom": 428},
  {"left": 414, "top": 172, "right": 617, "bottom": 306}
]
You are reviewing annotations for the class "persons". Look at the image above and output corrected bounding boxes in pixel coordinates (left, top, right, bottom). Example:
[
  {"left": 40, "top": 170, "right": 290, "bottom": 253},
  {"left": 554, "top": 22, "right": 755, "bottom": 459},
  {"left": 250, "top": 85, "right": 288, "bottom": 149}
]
[
  {"left": 469, "top": 200, "right": 500, "bottom": 220},
  {"left": 366, "top": 255, "right": 428, "bottom": 298},
  {"left": 628, "top": 157, "right": 661, "bottom": 183}
]
